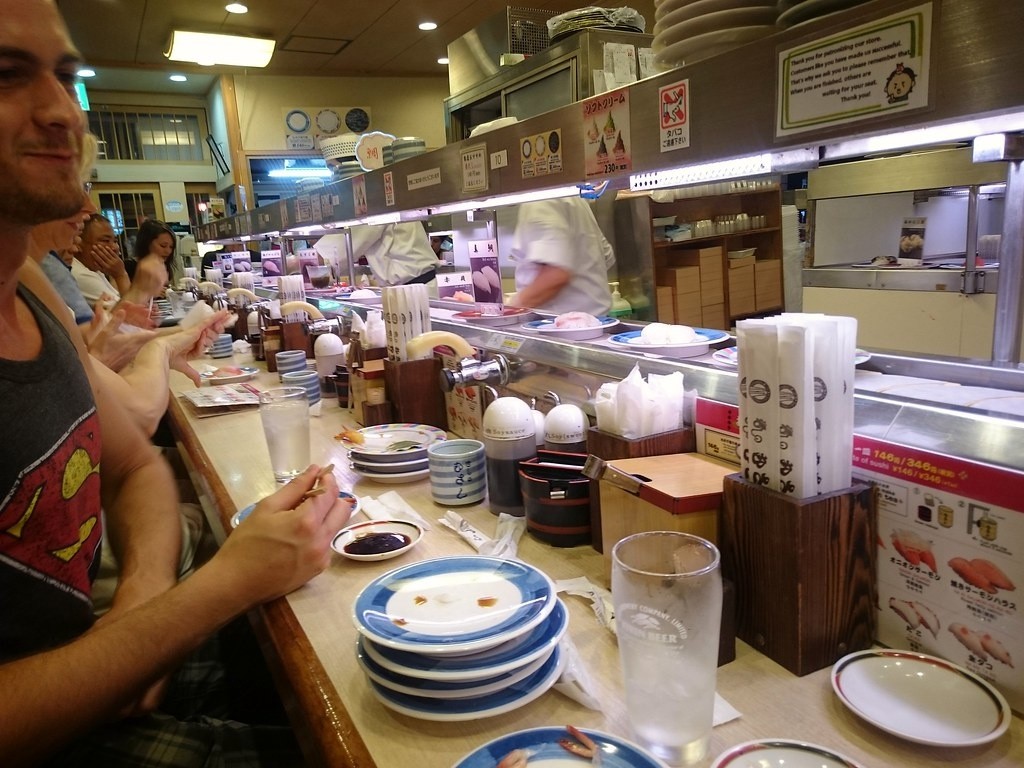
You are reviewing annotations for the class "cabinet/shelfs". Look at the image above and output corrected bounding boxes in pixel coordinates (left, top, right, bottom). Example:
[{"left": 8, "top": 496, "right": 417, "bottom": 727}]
[{"left": 614, "top": 178, "right": 783, "bottom": 330}]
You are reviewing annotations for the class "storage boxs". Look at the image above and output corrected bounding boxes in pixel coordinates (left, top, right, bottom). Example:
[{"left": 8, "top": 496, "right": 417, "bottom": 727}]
[
  {"left": 722, "top": 471, "right": 877, "bottom": 679},
  {"left": 383, "top": 352, "right": 451, "bottom": 432},
  {"left": 587, "top": 423, "right": 693, "bottom": 556},
  {"left": 279, "top": 320, "right": 314, "bottom": 357},
  {"left": 500, "top": 54, "right": 534, "bottom": 66},
  {"left": 638, "top": 246, "right": 782, "bottom": 333}
]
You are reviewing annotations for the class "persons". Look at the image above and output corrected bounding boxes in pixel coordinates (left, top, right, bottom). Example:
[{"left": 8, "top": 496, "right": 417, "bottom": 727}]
[
  {"left": 0, "top": 0, "right": 352, "bottom": 768},
  {"left": 310, "top": 221, "right": 439, "bottom": 287},
  {"left": 201, "top": 245, "right": 262, "bottom": 277},
  {"left": 29, "top": 130, "right": 231, "bottom": 615},
  {"left": 108, "top": 220, "right": 176, "bottom": 292},
  {"left": 507, "top": 197, "right": 616, "bottom": 316},
  {"left": 71, "top": 215, "right": 133, "bottom": 309},
  {"left": 431, "top": 235, "right": 448, "bottom": 257}
]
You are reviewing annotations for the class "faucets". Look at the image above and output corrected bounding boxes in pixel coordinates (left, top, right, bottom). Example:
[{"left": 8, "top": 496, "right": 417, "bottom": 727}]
[
  {"left": 178, "top": 277, "right": 201, "bottom": 295},
  {"left": 280, "top": 300, "right": 343, "bottom": 336},
  {"left": 405, "top": 331, "right": 509, "bottom": 400},
  {"left": 197, "top": 281, "right": 228, "bottom": 308},
  {"left": 226, "top": 288, "right": 271, "bottom": 311}
]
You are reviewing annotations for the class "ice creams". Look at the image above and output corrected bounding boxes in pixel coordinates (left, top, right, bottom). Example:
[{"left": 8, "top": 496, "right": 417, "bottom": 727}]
[{"left": 589, "top": 110, "right": 625, "bottom": 163}]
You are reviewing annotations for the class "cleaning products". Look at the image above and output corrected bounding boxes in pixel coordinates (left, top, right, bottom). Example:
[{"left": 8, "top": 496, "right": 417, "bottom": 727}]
[
  {"left": 608, "top": 281, "right": 632, "bottom": 320},
  {"left": 625, "top": 277, "right": 652, "bottom": 321}
]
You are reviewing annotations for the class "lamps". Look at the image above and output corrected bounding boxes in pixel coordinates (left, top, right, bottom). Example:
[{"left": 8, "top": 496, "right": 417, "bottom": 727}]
[
  {"left": 160, "top": 26, "right": 274, "bottom": 67},
  {"left": 268, "top": 167, "right": 333, "bottom": 177}
]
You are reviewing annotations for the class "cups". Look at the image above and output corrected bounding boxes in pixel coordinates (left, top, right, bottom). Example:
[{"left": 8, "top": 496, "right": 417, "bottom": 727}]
[
  {"left": 613, "top": 529, "right": 724, "bottom": 766},
  {"left": 295, "top": 139, "right": 427, "bottom": 195},
  {"left": 209, "top": 333, "right": 232, "bottom": 358},
  {"left": 275, "top": 350, "right": 307, "bottom": 383},
  {"left": 686, "top": 213, "right": 767, "bottom": 237},
  {"left": 427, "top": 439, "right": 486, "bottom": 505},
  {"left": 281, "top": 370, "right": 321, "bottom": 407},
  {"left": 260, "top": 387, "right": 310, "bottom": 484}
]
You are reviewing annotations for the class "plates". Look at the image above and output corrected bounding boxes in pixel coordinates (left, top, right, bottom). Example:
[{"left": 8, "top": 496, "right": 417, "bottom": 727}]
[
  {"left": 341, "top": 424, "right": 448, "bottom": 483},
  {"left": 550, "top": 12, "right": 643, "bottom": 44},
  {"left": 229, "top": 492, "right": 360, "bottom": 529},
  {"left": 452, "top": 725, "right": 671, "bottom": 768},
  {"left": 651, "top": 0, "right": 870, "bottom": 70},
  {"left": 710, "top": 739, "right": 866, "bottom": 768},
  {"left": 330, "top": 519, "right": 424, "bottom": 561},
  {"left": 303, "top": 289, "right": 382, "bottom": 305},
  {"left": 159, "top": 317, "right": 183, "bottom": 327},
  {"left": 831, "top": 648, "right": 1011, "bottom": 746},
  {"left": 351, "top": 553, "right": 568, "bottom": 722},
  {"left": 451, "top": 309, "right": 872, "bottom": 366},
  {"left": 199, "top": 367, "right": 260, "bottom": 384},
  {"left": 154, "top": 300, "right": 171, "bottom": 309},
  {"left": 853, "top": 262, "right": 999, "bottom": 269}
]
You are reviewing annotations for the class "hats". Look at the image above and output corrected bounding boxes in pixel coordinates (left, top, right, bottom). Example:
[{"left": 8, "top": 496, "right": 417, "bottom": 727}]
[
  {"left": 469, "top": 117, "right": 518, "bottom": 138},
  {"left": 319, "top": 134, "right": 361, "bottom": 161}
]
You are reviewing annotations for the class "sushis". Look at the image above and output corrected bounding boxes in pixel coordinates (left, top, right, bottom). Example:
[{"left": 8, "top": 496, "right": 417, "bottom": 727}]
[
  {"left": 349, "top": 288, "right": 377, "bottom": 299},
  {"left": 554, "top": 311, "right": 604, "bottom": 330},
  {"left": 453, "top": 291, "right": 474, "bottom": 302},
  {"left": 889, "top": 533, "right": 1016, "bottom": 668},
  {"left": 224, "top": 262, "right": 252, "bottom": 272},
  {"left": 213, "top": 366, "right": 246, "bottom": 376},
  {"left": 472, "top": 266, "right": 501, "bottom": 293},
  {"left": 263, "top": 261, "right": 280, "bottom": 273},
  {"left": 641, "top": 321, "right": 700, "bottom": 348}
]
